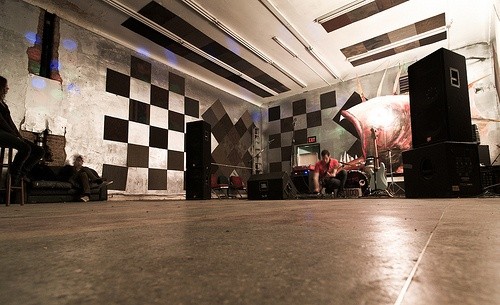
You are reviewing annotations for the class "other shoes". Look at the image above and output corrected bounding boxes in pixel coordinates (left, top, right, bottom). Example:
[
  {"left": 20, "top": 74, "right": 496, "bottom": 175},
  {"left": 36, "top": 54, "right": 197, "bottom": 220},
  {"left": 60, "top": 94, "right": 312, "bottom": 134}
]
[
  {"left": 99, "top": 180, "right": 113, "bottom": 188},
  {"left": 80, "top": 196, "right": 89, "bottom": 202}
]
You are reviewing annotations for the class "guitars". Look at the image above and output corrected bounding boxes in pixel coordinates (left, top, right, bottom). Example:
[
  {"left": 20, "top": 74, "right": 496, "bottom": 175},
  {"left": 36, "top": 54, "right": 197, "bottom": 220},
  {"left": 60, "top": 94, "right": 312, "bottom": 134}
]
[{"left": 370, "top": 127, "right": 389, "bottom": 191}]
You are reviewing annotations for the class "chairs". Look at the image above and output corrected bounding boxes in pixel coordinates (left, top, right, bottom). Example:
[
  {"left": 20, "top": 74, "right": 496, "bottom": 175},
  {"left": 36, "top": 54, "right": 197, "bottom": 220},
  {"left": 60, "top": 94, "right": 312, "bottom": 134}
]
[
  {"left": 211, "top": 175, "right": 229, "bottom": 200},
  {"left": 216, "top": 176, "right": 237, "bottom": 200},
  {"left": 229, "top": 176, "right": 247, "bottom": 199}
]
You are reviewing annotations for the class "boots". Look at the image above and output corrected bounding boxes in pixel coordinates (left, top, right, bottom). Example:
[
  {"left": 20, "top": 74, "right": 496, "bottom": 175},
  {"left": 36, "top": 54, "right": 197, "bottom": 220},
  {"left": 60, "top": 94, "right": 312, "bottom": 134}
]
[
  {"left": 22, "top": 154, "right": 40, "bottom": 187},
  {"left": 10, "top": 158, "right": 26, "bottom": 190}
]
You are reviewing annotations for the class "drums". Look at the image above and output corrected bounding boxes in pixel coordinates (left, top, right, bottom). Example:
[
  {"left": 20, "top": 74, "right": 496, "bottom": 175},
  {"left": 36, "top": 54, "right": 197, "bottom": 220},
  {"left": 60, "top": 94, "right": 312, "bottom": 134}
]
[
  {"left": 344, "top": 170, "right": 370, "bottom": 196},
  {"left": 364, "top": 156, "right": 374, "bottom": 168}
]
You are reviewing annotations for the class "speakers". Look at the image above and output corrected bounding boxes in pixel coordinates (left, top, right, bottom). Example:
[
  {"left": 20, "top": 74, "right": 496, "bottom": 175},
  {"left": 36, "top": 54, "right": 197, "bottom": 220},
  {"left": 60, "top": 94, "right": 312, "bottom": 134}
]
[
  {"left": 247, "top": 171, "right": 297, "bottom": 199},
  {"left": 293, "top": 169, "right": 322, "bottom": 193},
  {"left": 402, "top": 143, "right": 482, "bottom": 199},
  {"left": 186, "top": 120, "right": 211, "bottom": 200},
  {"left": 408, "top": 48, "right": 472, "bottom": 148}
]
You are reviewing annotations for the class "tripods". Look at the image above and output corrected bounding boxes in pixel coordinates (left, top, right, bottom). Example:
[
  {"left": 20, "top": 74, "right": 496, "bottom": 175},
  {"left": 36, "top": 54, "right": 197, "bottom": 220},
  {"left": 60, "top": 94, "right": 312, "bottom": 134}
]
[
  {"left": 387, "top": 151, "right": 405, "bottom": 196},
  {"left": 357, "top": 139, "right": 394, "bottom": 198}
]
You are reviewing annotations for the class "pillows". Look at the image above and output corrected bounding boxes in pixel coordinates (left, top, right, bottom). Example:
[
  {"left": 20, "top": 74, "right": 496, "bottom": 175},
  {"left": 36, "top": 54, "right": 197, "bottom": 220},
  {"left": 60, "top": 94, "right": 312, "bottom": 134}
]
[
  {"left": 27, "top": 161, "right": 56, "bottom": 182},
  {"left": 56, "top": 164, "right": 74, "bottom": 182}
]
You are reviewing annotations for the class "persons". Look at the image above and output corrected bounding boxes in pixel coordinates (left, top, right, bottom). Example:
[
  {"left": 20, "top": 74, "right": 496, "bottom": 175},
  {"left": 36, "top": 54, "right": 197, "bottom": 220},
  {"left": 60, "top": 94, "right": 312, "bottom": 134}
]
[
  {"left": 313, "top": 150, "right": 348, "bottom": 194},
  {"left": 70, "top": 155, "right": 114, "bottom": 203},
  {"left": 0, "top": 76, "right": 45, "bottom": 188}
]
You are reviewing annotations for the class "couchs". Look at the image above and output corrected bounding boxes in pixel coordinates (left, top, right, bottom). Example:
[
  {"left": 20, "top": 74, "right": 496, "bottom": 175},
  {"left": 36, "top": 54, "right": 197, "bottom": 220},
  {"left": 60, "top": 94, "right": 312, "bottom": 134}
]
[{"left": 5, "top": 165, "right": 108, "bottom": 203}]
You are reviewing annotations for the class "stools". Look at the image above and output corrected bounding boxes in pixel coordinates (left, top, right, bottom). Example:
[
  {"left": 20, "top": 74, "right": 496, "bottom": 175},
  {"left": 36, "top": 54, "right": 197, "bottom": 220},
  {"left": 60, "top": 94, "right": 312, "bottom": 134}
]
[{"left": 0, "top": 142, "right": 24, "bottom": 206}]
